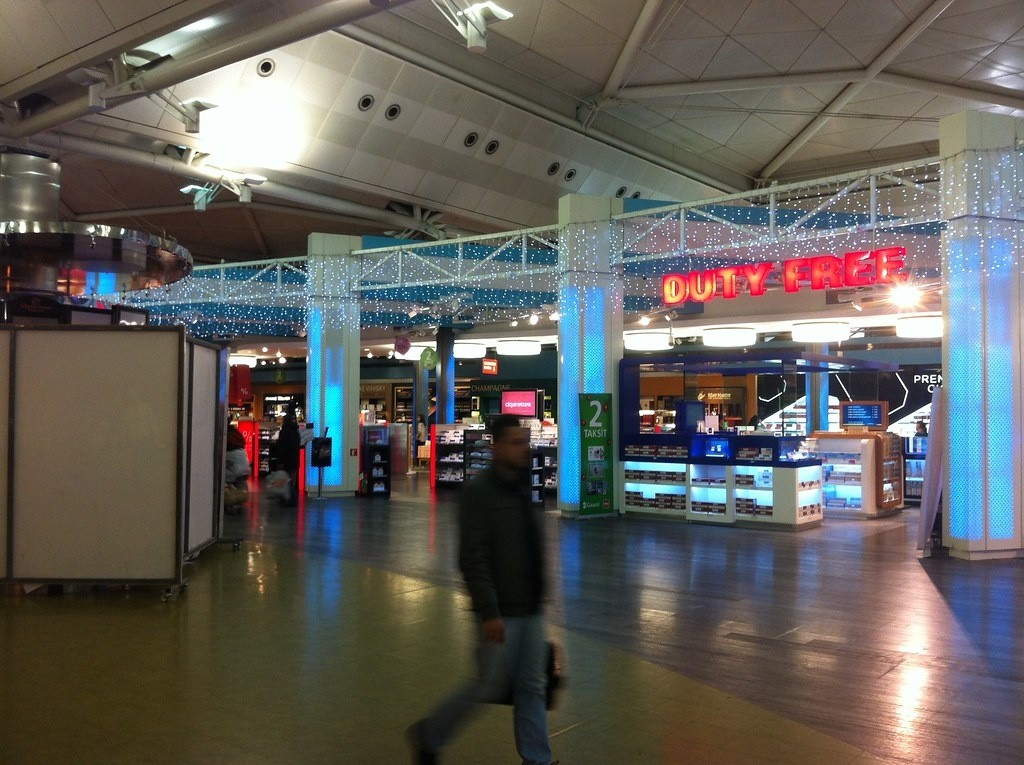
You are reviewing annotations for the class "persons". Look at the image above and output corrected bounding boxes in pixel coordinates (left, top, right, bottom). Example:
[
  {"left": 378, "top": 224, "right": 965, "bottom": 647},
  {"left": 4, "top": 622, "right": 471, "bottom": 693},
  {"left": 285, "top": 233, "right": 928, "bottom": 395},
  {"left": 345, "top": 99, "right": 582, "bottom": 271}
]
[
  {"left": 404, "top": 413, "right": 563, "bottom": 765},
  {"left": 225, "top": 415, "right": 252, "bottom": 485},
  {"left": 914, "top": 421, "right": 928, "bottom": 436},
  {"left": 415, "top": 414, "right": 426, "bottom": 466},
  {"left": 276, "top": 414, "right": 301, "bottom": 506}
]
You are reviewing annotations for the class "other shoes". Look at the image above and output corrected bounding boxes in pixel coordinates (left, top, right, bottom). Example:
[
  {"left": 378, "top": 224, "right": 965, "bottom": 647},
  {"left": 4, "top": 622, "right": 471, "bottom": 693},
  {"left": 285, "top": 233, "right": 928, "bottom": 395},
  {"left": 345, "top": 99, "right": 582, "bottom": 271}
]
[
  {"left": 405, "top": 719, "right": 439, "bottom": 765},
  {"left": 522, "top": 759, "right": 560, "bottom": 765}
]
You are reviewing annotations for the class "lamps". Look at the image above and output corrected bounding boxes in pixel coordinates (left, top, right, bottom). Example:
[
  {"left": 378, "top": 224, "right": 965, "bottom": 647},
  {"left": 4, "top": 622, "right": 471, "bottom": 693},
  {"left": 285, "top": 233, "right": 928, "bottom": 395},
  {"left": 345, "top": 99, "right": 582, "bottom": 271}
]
[
  {"left": 424, "top": 223, "right": 447, "bottom": 240},
  {"left": 383, "top": 227, "right": 414, "bottom": 239},
  {"left": 219, "top": 173, "right": 266, "bottom": 203},
  {"left": 429, "top": 0, "right": 512, "bottom": 51},
  {"left": 156, "top": 87, "right": 219, "bottom": 133},
  {"left": 180, "top": 182, "right": 223, "bottom": 211}
]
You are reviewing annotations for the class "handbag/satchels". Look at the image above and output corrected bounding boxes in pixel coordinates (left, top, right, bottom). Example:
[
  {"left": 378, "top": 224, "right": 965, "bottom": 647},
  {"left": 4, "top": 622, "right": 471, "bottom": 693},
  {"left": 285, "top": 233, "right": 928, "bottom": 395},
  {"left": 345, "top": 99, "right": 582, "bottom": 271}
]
[{"left": 497, "top": 641, "right": 560, "bottom": 710}]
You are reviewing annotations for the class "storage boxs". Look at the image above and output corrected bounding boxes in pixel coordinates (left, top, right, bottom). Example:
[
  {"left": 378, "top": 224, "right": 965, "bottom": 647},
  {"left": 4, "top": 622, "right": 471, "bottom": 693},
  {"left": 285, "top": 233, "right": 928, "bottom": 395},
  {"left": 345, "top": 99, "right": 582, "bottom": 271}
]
[
  {"left": 624, "top": 436, "right": 900, "bottom": 517},
  {"left": 438, "top": 417, "right": 557, "bottom": 502}
]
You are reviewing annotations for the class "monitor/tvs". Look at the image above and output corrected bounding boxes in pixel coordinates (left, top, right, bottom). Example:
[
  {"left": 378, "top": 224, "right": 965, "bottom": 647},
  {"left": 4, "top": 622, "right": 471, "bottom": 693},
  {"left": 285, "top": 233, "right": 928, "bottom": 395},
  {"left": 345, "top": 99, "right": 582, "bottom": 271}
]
[
  {"left": 840, "top": 401, "right": 889, "bottom": 431},
  {"left": 682, "top": 401, "right": 705, "bottom": 431},
  {"left": 537, "top": 390, "right": 545, "bottom": 421},
  {"left": 500, "top": 388, "right": 538, "bottom": 417}
]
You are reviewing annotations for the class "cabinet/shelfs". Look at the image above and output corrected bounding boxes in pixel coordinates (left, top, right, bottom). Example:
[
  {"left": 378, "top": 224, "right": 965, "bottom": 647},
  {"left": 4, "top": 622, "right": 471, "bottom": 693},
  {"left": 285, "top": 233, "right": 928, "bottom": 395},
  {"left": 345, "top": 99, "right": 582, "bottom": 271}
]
[
  {"left": 806, "top": 431, "right": 903, "bottom": 519},
  {"left": 901, "top": 437, "right": 930, "bottom": 505},
  {"left": 430, "top": 423, "right": 557, "bottom": 507},
  {"left": 359, "top": 443, "right": 390, "bottom": 499},
  {"left": 256, "top": 427, "right": 281, "bottom": 475},
  {"left": 616, "top": 432, "right": 823, "bottom": 532}
]
